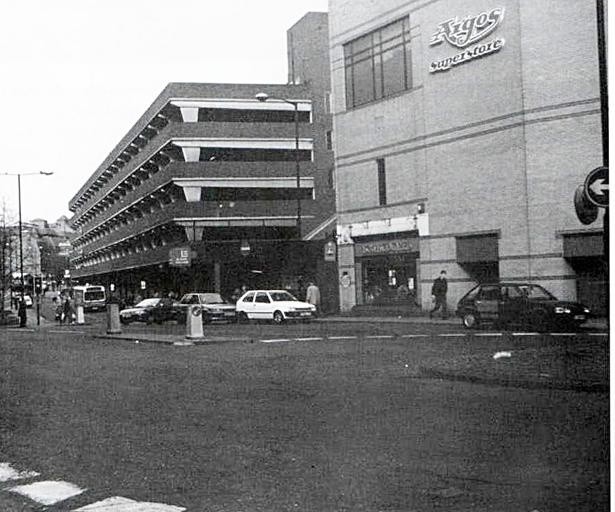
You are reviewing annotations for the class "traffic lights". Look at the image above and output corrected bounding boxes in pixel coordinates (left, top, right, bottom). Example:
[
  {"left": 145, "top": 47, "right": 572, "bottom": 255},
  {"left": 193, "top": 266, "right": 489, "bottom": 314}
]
[{"left": 36, "top": 278, "right": 42, "bottom": 293}]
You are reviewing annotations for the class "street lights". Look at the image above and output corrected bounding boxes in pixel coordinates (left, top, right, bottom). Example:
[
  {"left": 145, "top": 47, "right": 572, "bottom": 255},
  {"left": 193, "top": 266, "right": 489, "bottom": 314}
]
[
  {"left": 256, "top": 94, "right": 302, "bottom": 238},
  {"left": 2, "top": 172, "right": 53, "bottom": 327}
]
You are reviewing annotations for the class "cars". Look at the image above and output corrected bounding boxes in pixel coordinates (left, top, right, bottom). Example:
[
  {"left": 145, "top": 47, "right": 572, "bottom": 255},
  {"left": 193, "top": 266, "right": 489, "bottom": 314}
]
[
  {"left": 455, "top": 283, "right": 589, "bottom": 332},
  {"left": 119, "top": 288, "right": 318, "bottom": 325},
  {"left": 19, "top": 295, "right": 34, "bottom": 307}
]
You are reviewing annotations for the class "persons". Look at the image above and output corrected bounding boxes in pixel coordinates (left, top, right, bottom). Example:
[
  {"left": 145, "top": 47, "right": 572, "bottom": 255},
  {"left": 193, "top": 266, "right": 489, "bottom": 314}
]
[
  {"left": 430, "top": 270, "right": 448, "bottom": 320},
  {"left": 53, "top": 291, "right": 77, "bottom": 325},
  {"left": 306, "top": 282, "right": 320, "bottom": 320}
]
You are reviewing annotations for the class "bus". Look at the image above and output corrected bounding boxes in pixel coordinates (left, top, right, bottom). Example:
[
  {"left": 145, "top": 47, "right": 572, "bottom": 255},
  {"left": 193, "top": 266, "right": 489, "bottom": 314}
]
[{"left": 67, "top": 286, "right": 106, "bottom": 312}]
